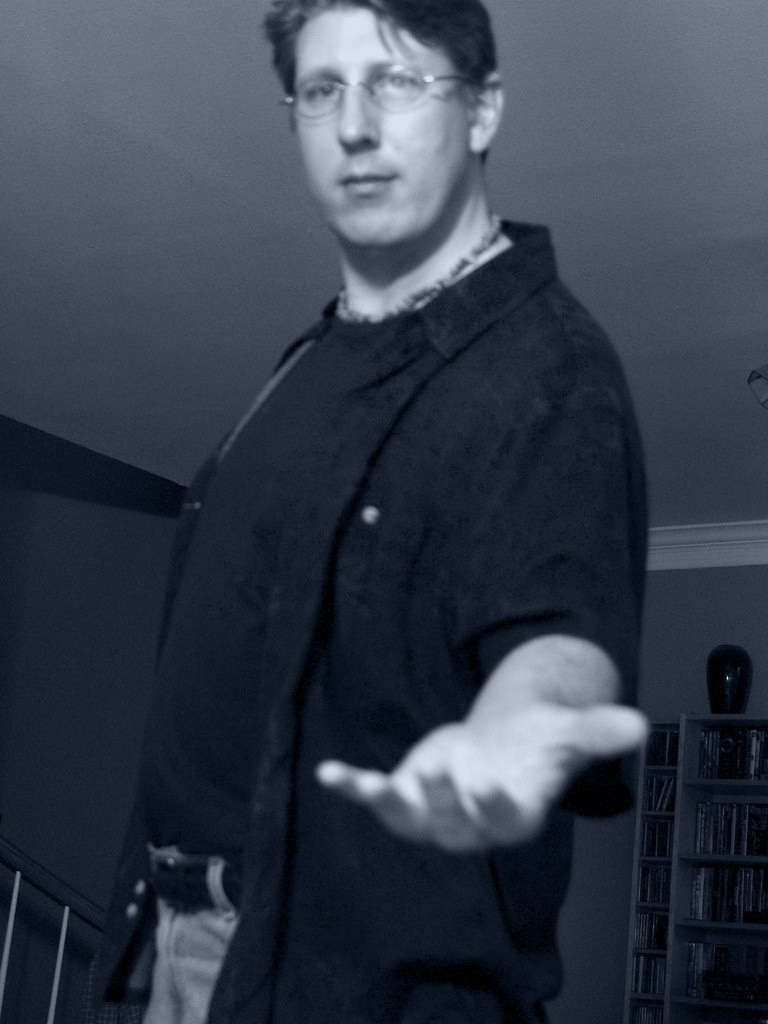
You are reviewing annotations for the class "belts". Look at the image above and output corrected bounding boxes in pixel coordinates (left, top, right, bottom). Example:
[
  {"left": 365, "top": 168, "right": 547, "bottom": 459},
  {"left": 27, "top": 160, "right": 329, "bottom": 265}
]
[{"left": 145, "top": 863, "right": 246, "bottom": 913}]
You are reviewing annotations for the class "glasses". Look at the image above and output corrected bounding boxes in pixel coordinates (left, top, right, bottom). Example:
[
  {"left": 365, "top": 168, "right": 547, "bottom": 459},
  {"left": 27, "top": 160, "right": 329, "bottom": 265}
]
[{"left": 277, "top": 66, "right": 476, "bottom": 119}]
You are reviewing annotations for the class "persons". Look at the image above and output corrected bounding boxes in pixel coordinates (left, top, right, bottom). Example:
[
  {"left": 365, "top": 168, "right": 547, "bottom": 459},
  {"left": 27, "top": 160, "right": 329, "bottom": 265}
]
[{"left": 89, "top": 0, "right": 645, "bottom": 1024}]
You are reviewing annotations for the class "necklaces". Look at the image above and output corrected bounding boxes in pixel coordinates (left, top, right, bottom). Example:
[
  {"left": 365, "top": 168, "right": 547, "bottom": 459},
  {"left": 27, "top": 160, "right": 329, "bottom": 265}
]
[{"left": 336, "top": 210, "right": 501, "bottom": 324}]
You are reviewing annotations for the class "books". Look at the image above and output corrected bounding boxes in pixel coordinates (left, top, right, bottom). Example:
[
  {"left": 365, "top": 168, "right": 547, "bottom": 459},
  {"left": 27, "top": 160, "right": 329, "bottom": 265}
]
[
  {"left": 688, "top": 867, "right": 768, "bottom": 923},
  {"left": 640, "top": 819, "right": 674, "bottom": 857},
  {"left": 643, "top": 775, "right": 675, "bottom": 812},
  {"left": 633, "top": 956, "right": 666, "bottom": 994},
  {"left": 630, "top": 1007, "right": 663, "bottom": 1024},
  {"left": 695, "top": 801, "right": 768, "bottom": 856},
  {"left": 685, "top": 941, "right": 767, "bottom": 1000},
  {"left": 698, "top": 729, "right": 768, "bottom": 779},
  {"left": 634, "top": 912, "right": 669, "bottom": 950},
  {"left": 637, "top": 866, "right": 670, "bottom": 904},
  {"left": 645, "top": 730, "right": 678, "bottom": 767}
]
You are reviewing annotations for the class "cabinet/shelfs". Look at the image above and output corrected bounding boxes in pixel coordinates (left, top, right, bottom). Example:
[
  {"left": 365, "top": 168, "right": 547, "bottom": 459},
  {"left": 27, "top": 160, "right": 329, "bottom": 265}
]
[{"left": 624, "top": 714, "right": 768, "bottom": 1024}]
